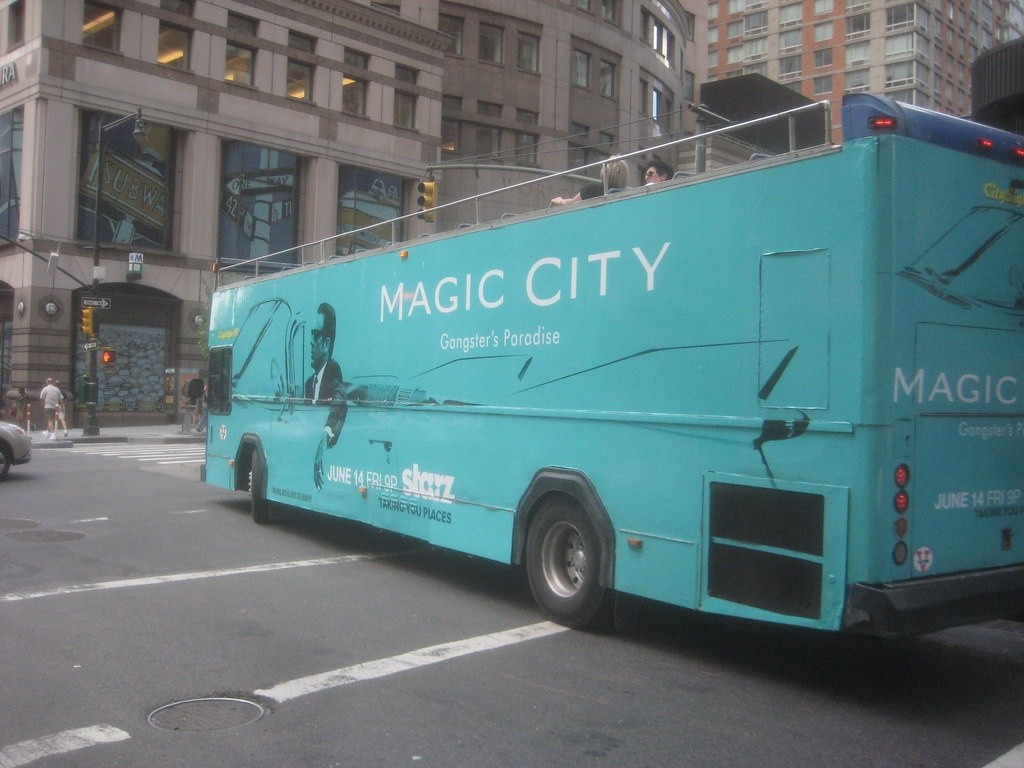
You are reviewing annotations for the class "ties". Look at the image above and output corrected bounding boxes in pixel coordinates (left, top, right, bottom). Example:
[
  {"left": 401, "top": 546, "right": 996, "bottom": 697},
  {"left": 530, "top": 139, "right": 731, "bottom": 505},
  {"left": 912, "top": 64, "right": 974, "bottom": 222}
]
[{"left": 312, "top": 376, "right": 318, "bottom": 399}]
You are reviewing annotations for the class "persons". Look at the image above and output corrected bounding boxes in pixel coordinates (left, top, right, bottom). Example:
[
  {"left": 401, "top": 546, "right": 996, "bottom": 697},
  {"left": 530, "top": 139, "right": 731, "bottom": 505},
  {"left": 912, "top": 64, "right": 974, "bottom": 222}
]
[
  {"left": 551, "top": 154, "right": 629, "bottom": 205},
  {"left": 643, "top": 158, "right": 674, "bottom": 186},
  {"left": 40, "top": 377, "right": 70, "bottom": 441},
  {"left": 305, "top": 302, "right": 347, "bottom": 492},
  {"left": 196, "top": 385, "right": 208, "bottom": 432}
]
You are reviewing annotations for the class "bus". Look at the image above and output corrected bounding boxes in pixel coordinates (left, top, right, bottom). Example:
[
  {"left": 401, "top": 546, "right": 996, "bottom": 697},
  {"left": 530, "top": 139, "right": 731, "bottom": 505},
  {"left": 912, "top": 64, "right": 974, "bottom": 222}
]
[{"left": 186, "top": 90, "right": 1024, "bottom": 644}]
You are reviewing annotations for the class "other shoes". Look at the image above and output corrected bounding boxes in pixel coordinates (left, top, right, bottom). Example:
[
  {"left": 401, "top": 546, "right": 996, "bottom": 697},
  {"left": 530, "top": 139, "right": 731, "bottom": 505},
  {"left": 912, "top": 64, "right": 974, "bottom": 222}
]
[
  {"left": 50, "top": 433, "right": 56, "bottom": 440},
  {"left": 43, "top": 430, "right": 52, "bottom": 437},
  {"left": 195, "top": 425, "right": 204, "bottom": 432},
  {"left": 63, "top": 430, "right": 68, "bottom": 437}
]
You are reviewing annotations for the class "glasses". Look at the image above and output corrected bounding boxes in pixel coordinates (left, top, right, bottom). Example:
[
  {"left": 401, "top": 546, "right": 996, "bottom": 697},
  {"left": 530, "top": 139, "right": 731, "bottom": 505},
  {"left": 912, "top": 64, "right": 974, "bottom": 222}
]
[
  {"left": 311, "top": 329, "right": 326, "bottom": 341},
  {"left": 644, "top": 170, "right": 657, "bottom": 177}
]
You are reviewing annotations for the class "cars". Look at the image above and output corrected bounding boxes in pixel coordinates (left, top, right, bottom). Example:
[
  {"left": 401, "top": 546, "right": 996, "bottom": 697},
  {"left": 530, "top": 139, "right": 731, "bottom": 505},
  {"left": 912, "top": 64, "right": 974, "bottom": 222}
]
[{"left": 0, "top": 420, "right": 32, "bottom": 481}]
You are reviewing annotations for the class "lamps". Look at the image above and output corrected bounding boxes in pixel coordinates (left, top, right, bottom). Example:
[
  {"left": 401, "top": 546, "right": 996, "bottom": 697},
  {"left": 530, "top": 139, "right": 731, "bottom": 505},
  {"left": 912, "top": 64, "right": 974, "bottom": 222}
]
[
  {"left": 194, "top": 314, "right": 205, "bottom": 327},
  {"left": 17, "top": 302, "right": 24, "bottom": 314},
  {"left": 46, "top": 303, "right": 58, "bottom": 316}
]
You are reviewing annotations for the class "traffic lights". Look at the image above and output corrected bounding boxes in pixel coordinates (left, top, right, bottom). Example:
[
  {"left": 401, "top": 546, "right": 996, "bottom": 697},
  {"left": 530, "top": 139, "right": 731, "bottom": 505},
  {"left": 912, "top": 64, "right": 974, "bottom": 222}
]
[
  {"left": 100, "top": 348, "right": 116, "bottom": 365},
  {"left": 417, "top": 181, "right": 436, "bottom": 223},
  {"left": 81, "top": 306, "right": 94, "bottom": 336}
]
